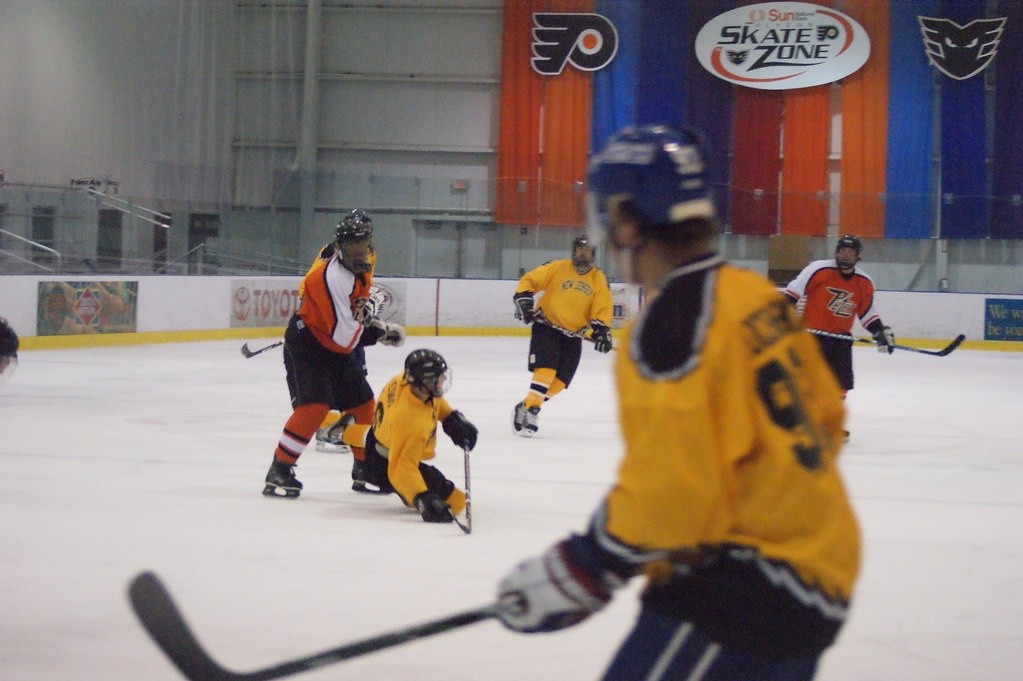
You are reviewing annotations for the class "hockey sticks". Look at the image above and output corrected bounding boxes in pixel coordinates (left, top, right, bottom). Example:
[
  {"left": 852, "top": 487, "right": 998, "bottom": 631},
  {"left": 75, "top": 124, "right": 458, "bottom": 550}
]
[
  {"left": 805, "top": 324, "right": 965, "bottom": 357},
  {"left": 240, "top": 342, "right": 283, "bottom": 358},
  {"left": 447, "top": 438, "right": 474, "bottom": 534},
  {"left": 515, "top": 312, "right": 620, "bottom": 352},
  {"left": 125, "top": 569, "right": 494, "bottom": 674}
]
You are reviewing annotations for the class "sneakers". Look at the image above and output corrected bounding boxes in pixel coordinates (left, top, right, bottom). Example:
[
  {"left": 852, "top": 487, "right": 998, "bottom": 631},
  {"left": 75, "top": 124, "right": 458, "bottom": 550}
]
[
  {"left": 264, "top": 458, "right": 303, "bottom": 489},
  {"left": 514, "top": 401, "right": 525, "bottom": 431},
  {"left": 522, "top": 406, "right": 541, "bottom": 432},
  {"left": 351, "top": 458, "right": 386, "bottom": 485},
  {"left": 315, "top": 428, "right": 347, "bottom": 445}
]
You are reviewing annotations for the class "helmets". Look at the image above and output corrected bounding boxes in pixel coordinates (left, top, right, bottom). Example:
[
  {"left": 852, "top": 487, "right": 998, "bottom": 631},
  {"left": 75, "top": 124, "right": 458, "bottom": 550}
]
[
  {"left": 585, "top": 122, "right": 716, "bottom": 231},
  {"left": 835, "top": 233, "right": 862, "bottom": 271},
  {"left": 571, "top": 233, "right": 596, "bottom": 269},
  {"left": 333, "top": 206, "right": 376, "bottom": 275}
]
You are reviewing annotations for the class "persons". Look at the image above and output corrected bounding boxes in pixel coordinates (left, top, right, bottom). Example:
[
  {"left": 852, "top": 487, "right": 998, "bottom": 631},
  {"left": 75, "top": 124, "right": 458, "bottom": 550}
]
[
  {"left": 513, "top": 235, "right": 613, "bottom": 432},
  {"left": 785, "top": 236, "right": 895, "bottom": 436},
  {"left": 495, "top": 122, "right": 895, "bottom": 681},
  {"left": 262, "top": 208, "right": 405, "bottom": 489},
  {"left": 329, "top": 349, "right": 478, "bottom": 522},
  {"left": 0, "top": 316, "right": 19, "bottom": 374}
]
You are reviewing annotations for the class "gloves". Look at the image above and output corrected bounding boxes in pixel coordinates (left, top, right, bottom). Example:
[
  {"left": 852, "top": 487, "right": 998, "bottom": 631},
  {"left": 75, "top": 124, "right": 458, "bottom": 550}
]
[
  {"left": 352, "top": 284, "right": 385, "bottom": 328},
  {"left": 512, "top": 292, "right": 545, "bottom": 326},
  {"left": 490, "top": 497, "right": 671, "bottom": 633},
  {"left": 413, "top": 491, "right": 453, "bottom": 524},
  {"left": 370, "top": 319, "right": 405, "bottom": 347},
  {"left": 590, "top": 319, "right": 613, "bottom": 353},
  {"left": 442, "top": 410, "right": 477, "bottom": 452},
  {"left": 873, "top": 325, "right": 895, "bottom": 354}
]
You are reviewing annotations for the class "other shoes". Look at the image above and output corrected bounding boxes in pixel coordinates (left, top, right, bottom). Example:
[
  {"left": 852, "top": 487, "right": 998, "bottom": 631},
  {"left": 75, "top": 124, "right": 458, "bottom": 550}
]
[{"left": 842, "top": 429, "right": 849, "bottom": 437}]
